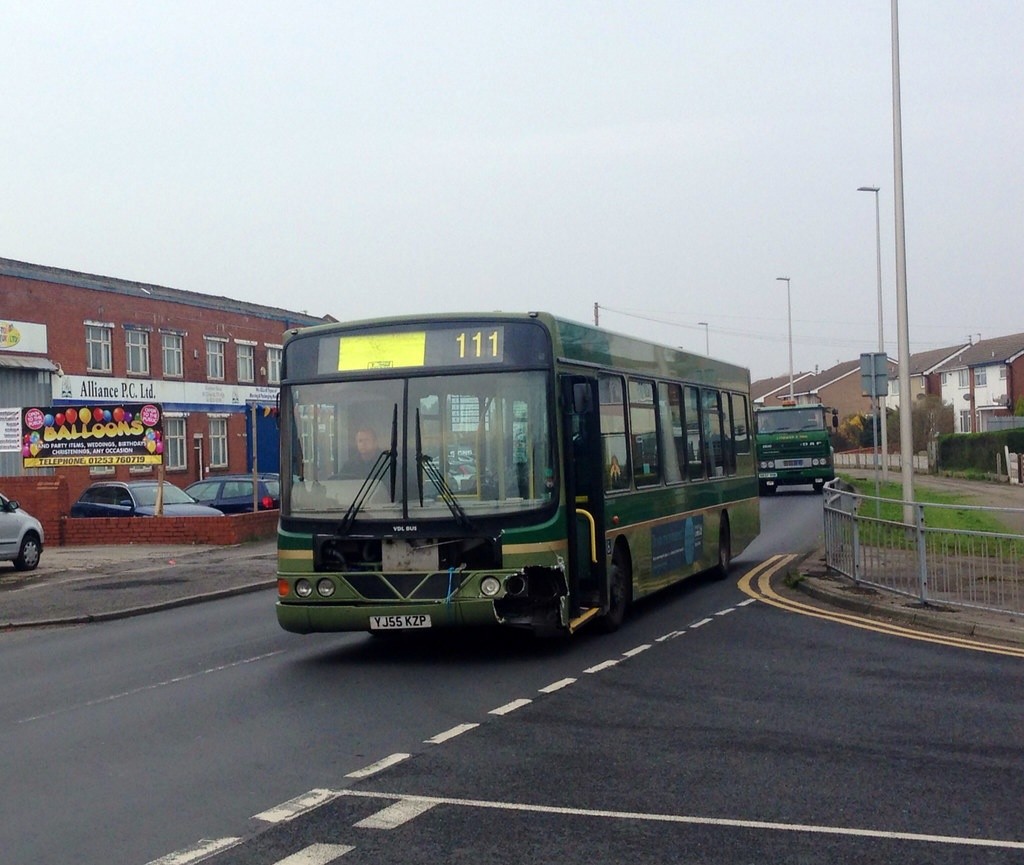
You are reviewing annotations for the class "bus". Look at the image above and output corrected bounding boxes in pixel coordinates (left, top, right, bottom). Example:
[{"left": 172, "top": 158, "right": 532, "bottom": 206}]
[{"left": 275, "top": 311, "right": 761, "bottom": 634}]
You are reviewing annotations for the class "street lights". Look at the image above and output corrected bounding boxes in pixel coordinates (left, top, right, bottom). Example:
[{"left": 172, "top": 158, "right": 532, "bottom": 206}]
[
  {"left": 858, "top": 186, "right": 890, "bottom": 480},
  {"left": 776, "top": 277, "right": 794, "bottom": 400},
  {"left": 698, "top": 322, "right": 710, "bottom": 357}
]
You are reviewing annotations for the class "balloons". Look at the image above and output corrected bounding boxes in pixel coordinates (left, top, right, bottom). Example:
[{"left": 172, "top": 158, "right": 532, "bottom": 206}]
[
  {"left": 44, "top": 408, "right": 140, "bottom": 427},
  {"left": 22, "top": 432, "right": 43, "bottom": 458},
  {"left": 142, "top": 427, "right": 162, "bottom": 454}
]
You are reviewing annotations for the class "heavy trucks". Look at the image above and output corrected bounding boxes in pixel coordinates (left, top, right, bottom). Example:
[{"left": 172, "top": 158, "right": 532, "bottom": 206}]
[{"left": 753, "top": 403, "right": 838, "bottom": 497}]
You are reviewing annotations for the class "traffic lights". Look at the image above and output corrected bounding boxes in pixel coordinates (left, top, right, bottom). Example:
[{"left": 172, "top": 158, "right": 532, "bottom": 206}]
[{"left": 543, "top": 467, "right": 555, "bottom": 490}]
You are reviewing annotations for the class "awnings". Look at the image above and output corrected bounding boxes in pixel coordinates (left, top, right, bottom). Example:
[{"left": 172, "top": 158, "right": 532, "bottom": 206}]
[{"left": 0, "top": 354, "right": 59, "bottom": 372}]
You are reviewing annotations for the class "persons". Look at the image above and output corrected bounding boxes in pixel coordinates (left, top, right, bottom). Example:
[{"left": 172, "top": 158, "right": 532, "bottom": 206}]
[{"left": 339, "top": 428, "right": 390, "bottom": 481}]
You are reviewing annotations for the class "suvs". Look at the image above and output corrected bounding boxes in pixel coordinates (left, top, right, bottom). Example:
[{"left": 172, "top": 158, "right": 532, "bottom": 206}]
[{"left": 70, "top": 479, "right": 224, "bottom": 517}]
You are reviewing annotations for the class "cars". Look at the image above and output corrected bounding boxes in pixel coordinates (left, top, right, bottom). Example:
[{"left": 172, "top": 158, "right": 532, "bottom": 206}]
[
  {"left": 182, "top": 477, "right": 273, "bottom": 514},
  {"left": 421, "top": 461, "right": 476, "bottom": 491},
  {"left": 0, "top": 492, "right": 45, "bottom": 571},
  {"left": 432, "top": 455, "right": 474, "bottom": 463}
]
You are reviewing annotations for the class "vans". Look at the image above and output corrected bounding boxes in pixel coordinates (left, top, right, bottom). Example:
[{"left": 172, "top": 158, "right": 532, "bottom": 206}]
[
  {"left": 619, "top": 437, "right": 688, "bottom": 485},
  {"left": 204, "top": 473, "right": 301, "bottom": 501}
]
